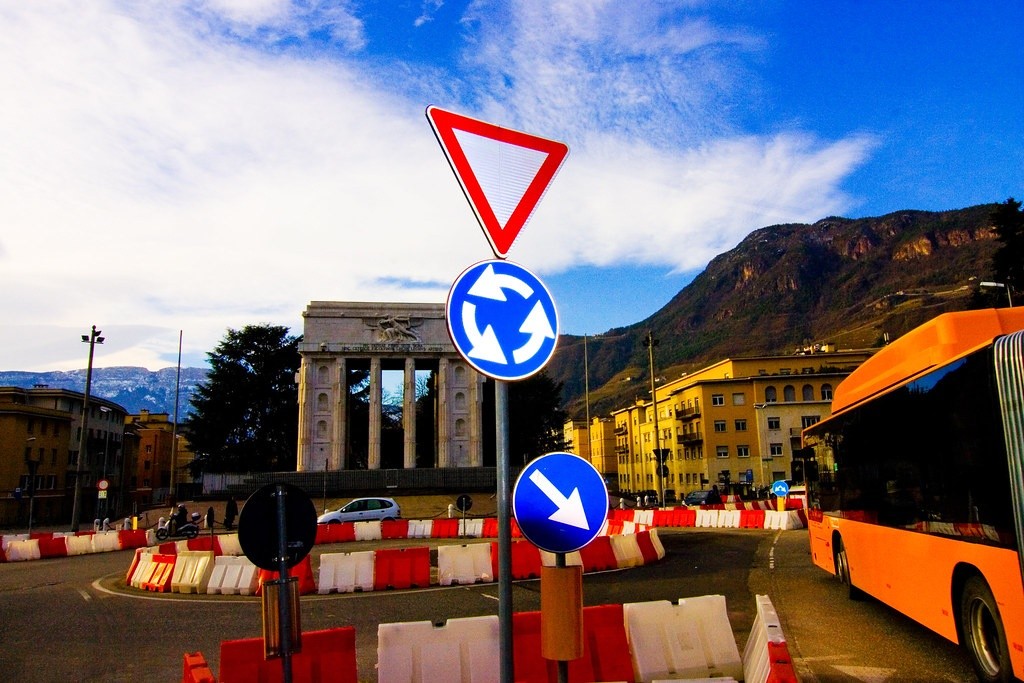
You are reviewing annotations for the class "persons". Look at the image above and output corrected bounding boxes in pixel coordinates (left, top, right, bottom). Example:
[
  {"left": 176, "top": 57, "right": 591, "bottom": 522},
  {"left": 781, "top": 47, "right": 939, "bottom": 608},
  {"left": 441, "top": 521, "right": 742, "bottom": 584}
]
[
  {"left": 225, "top": 493, "right": 238, "bottom": 530},
  {"left": 379, "top": 314, "right": 422, "bottom": 343},
  {"left": 172, "top": 502, "right": 188, "bottom": 535},
  {"left": 614, "top": 481, "right": 772, "bottom": 511}
]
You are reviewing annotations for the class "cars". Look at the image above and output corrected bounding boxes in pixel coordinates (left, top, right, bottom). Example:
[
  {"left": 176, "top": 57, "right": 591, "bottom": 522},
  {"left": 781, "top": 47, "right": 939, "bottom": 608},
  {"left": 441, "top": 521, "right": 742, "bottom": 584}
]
[{"left": 317, "top": 497, "right": 401, "bottom": 523}]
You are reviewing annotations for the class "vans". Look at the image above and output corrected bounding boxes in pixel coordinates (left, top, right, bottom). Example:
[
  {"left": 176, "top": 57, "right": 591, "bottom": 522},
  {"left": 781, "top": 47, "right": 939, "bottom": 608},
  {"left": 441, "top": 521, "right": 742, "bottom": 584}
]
[{"left": 682, "top": 491, "right": 715, "bottom": 504}]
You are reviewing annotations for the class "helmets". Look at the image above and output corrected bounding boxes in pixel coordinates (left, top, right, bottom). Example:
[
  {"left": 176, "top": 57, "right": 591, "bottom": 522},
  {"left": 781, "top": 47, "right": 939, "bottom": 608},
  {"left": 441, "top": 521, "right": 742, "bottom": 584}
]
[{"left": 178, "top": 504, "right": 185, "bottom": 510}]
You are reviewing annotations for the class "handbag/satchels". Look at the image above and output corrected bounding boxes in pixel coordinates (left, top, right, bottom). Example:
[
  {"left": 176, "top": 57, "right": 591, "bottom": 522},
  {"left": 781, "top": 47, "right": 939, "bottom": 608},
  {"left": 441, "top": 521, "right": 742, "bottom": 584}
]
[{"left": 223, "top": 516, "right": 228, "bottom": 526}]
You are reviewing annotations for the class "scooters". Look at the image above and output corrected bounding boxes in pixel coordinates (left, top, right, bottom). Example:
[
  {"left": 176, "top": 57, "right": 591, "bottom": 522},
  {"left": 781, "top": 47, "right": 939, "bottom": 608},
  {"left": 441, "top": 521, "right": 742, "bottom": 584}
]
[{"left": 157, "top": 512, "right": 201, "bottom": 539}]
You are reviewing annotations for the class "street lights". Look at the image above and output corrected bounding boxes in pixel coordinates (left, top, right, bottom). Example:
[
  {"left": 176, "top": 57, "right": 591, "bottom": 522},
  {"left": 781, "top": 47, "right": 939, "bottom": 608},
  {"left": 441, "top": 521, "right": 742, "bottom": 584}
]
[
  {"left": 71, "top": 326, "right": 104, "bottom": 532},
  {"left": 642, "top": 330, "right": 663, "bottom": 506},
  {"left": 26, "top": 438, "right": 36, "bottom": 533}
]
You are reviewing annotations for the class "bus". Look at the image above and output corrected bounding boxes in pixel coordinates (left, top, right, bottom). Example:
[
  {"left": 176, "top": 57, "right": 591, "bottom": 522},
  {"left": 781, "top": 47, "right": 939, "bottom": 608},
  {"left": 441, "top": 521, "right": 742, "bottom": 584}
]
[{"left": 791, "top": 306, "right": 1024, "bottom": 683}]
